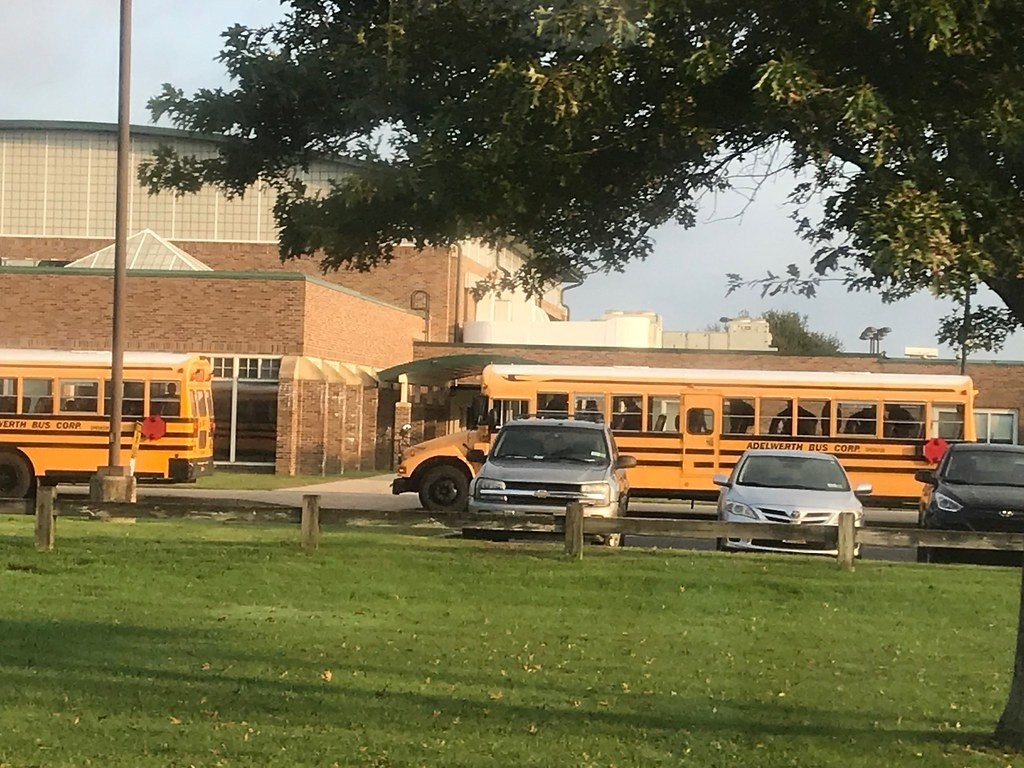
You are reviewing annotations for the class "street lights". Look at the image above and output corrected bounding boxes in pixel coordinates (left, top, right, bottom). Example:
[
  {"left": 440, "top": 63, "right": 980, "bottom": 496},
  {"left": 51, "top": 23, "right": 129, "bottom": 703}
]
[{"left": 859, "top": 326, "right": 891, "bottom": 354}]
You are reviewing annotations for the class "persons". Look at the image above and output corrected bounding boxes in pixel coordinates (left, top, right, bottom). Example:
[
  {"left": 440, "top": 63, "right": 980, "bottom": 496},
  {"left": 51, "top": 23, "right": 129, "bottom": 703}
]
[
  {"left": 541, "top": 394, "right": 604, "bottom": 424},
  {"left": 611, "top": 398, "right": 643, "bottom": 431},
  {"left": 688, "top": 398, "right": 919, "bottom": 438},
  {"left": 161, "top": 383, "right": 181, "bottom": 416}
]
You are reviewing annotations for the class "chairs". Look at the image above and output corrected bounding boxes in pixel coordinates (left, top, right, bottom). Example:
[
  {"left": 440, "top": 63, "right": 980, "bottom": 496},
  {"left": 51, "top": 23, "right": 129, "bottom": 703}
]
[
  {"left": 729, "top": 419, "right": 748, "bottom": 433},
  {"left": 653, "top": 414, "right": 667, "bottom": 432},
  {"left": 956, "top": 457, "right": 977, "bottom": 482},
  {"left": 769, "top": 419, "right": 781, "bottom": 434},
  {"left": 0, "top": 396, "right": 179, "bottom": 416},
  {"left": 516, "top": 438, "right": 543, "bottom": 458},
  {"left": 572, "top": 439, "right": 600, "bottom": 458},
  {"left": 1010, "top": 463, "right": 1024, "bottom": 484},
  {"left": 915, "top": 423, "right": 925, "bottom": 439},
  {"left": 955, "top": 423, "right": 965, "bottom": 440}
]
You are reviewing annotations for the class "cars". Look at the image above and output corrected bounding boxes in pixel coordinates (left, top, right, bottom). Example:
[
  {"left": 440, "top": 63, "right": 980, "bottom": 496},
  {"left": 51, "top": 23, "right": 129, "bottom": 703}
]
[
  {"left": 912, "top": 443, "right": 1024, "bottom": 568},
  {"left": 714, "top": 450, "right": 872, "bottom": 560}
]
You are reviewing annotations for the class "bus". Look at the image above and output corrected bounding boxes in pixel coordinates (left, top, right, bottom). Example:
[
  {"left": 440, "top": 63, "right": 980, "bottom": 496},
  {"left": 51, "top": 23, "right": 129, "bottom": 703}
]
[
  {"left": 0, "top": 346, "right": 215, "bottom": 495},
  {"left": 211, "top": 383, "right": 279, "bottom": 460},
  {"left": 390, "top": 363, "right": 981, "bottom": 517}
]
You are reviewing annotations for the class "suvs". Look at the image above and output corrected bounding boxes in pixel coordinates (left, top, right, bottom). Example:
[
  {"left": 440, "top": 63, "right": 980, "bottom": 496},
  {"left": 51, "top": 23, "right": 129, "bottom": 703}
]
[{"left": 463, "top": 419, "right": 637, "bottom": 546}]
